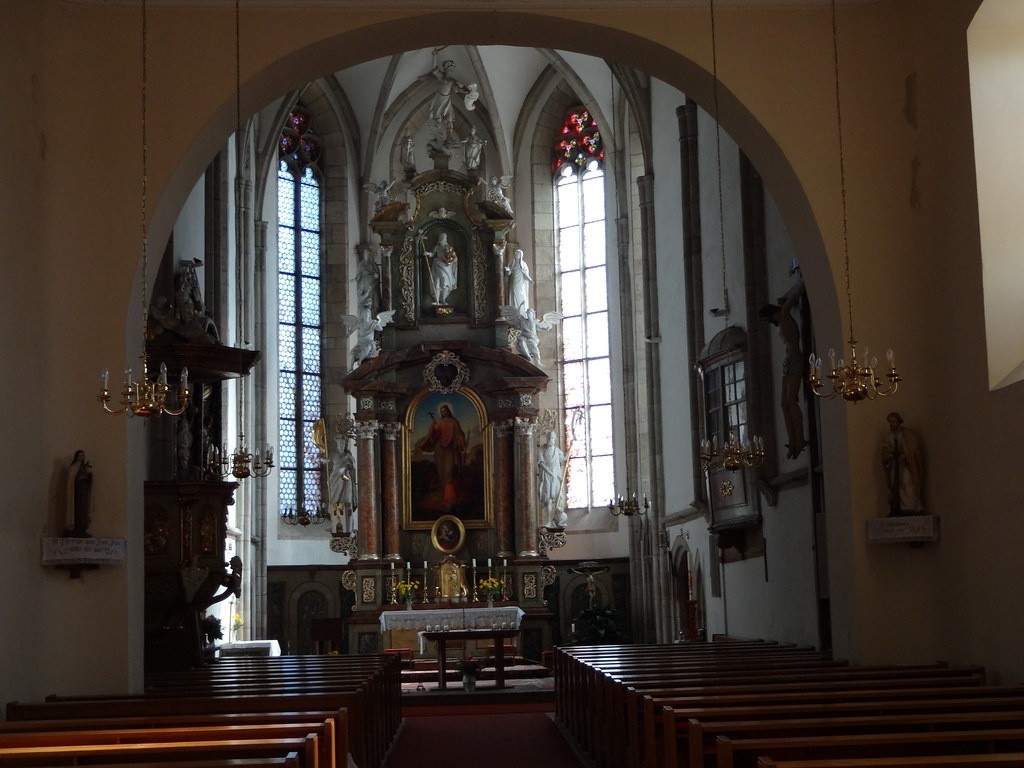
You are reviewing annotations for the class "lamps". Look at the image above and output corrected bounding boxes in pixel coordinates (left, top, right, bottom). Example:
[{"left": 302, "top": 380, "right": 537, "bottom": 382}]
[
  {"left": 280, "top": 80, "right": 329, "bottom": 527},
  {"left": 808, "top": 0, "right": 902, "bottom": 405},
  {"left": 202, "top": 0, "right": 275, "bottom": 480},
  {"left": 698, "top": 0, "right": 765, "bottom": 473},
  {"left": 608, "top": 57, "right": 650, "bottom": 517},
  {"left": 98, "top": 1, "right": 192, "bottom": 420}
]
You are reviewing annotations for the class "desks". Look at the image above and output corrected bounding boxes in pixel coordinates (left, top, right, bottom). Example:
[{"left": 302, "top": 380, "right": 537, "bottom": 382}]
[
  {"left": 417, "top": 628, "right": 520, "bottom": 693},
  {"left": 379, "top": 605, "right": 527, "bottom": 658}
]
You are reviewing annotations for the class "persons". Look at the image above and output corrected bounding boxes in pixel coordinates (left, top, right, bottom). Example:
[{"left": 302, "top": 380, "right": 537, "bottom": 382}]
[
  {"left": 202, "top": 416, "right": 213, "bottom": 467},
  {"left": 394, "top": 128, "right": 415, "bottom": 170},
  {"left": 375, "top": 178, "right": 397, "bottom": 208},
  {"left": 571, "top": 567, "right": 607, "bottom": 610},
  {"left": 460, "top": 126, "right": 485, "bottom": 169},
  {"left": 504, "top": 250, "right": 532, "bottom": 317},
  {"left": 882, "top": 410, "right": 924, "bottom": 515},
  {"left": 517, "top": 299, "right": 549, "bottom": 369},
  {"left": 346, "top": 309, "right": 383, "bottom": 373},
  {"left": 422, "top": 233, "right": 458, "bottom": 305},
  {"left": 537, "top": 432, "right": 571, "bottom": 528},
  {"left": 354, "top": 250, "right": 382, "bottom": 317},
  {"left": 479, "top": 175, "right": 514, "bottom": 214},
  {"left": 179, "top": 416, "right": 190, "bottom": 474},
  {"left": 431, "top": 48, "right": 468, "bottom": 132},
  {"left": 759, "top": 281, "right": 810, "bottom": 460},
  {"left": 320, "top": 436, "right": 359, "bottom": 532},
  {"left": 64, "top": 449, "right": 96, "bottom": 537}
]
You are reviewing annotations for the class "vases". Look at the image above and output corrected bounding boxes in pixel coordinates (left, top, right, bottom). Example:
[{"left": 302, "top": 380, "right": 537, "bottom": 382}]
[
  {"left": 405, "top": 595, "right": 414, "bottom": 612},
  {"left": 462, "top": 673, "right": 476, "bottom": 692},
  {"left": 486, "top": 593, "right": 494, "bottom": 608}
]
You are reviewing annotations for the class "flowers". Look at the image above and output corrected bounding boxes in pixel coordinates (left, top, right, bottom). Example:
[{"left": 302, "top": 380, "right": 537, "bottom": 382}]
[
  {"left": 456, "top": 656, "right": 478, "bottom": 674},
  {"left": 396, "top": 578, "right": 421, "bottom": 597},
  {"left": 478, "top": 578, "right": 507, "bottom": 600}
]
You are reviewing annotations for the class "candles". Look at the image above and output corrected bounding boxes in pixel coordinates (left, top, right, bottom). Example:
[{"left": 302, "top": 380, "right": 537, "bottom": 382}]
[
  {"left": 424, "top": 560, "right": 428, "bottom": 568},
  {"left": 391, "top": 561, "right": 394, "bottom": 570},
  {"left": 488, "top": 558, "right": 491, "bottom": 566},
  {"left": 503, "top": 559, "right": 507, "bottom": 566},
  {"left": 407, "top": 562, "right": 411, "bottom": 568},
  {"left": 472, "top": 558, "right": 477, "bottom": 567},
  {"left": 426, "top": 622, "right": 516, "bottom": 632}
]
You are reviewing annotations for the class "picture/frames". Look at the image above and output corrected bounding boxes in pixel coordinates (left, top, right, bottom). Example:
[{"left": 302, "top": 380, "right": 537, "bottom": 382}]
[
  {"left": 401, "top": 384, "right": 495, "bottom": 532},
  {"left": 430, "top": 514, "right": 466, "bottom": 553}
]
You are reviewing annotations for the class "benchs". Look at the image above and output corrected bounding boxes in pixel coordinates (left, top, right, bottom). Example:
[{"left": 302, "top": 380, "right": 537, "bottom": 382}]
[
  {"left": 0, "top": 653, "right": 405, "bottom": 768},
  {"left": 554, "top": 639, "right": 1024, "bottom": 768}
]
